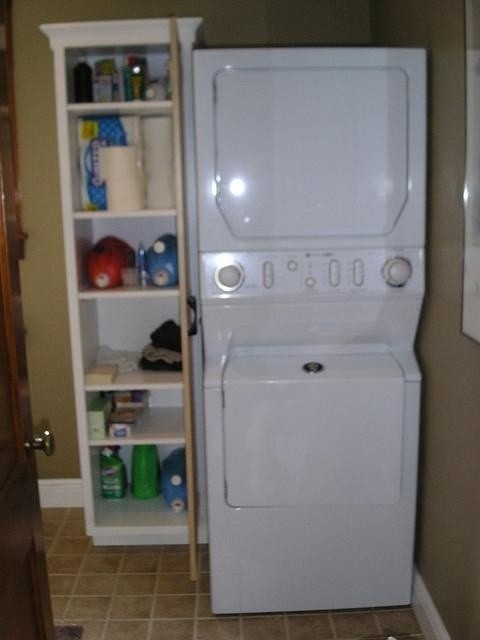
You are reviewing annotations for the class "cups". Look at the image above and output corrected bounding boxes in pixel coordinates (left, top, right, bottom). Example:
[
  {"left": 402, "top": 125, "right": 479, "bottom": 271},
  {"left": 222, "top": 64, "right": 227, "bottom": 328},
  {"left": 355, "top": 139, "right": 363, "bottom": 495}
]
[{"left": 120, "top": 268, "right": 140, "bottom": 289}]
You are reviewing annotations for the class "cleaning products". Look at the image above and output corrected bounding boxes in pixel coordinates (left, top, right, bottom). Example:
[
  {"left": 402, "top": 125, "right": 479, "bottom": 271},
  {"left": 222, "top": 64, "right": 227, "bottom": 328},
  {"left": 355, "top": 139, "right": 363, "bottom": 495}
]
[
  {"left": 161, "top": 447, "right": 186, "bottom": 513},
  {"left": 85, "top": 234, "right": 137, "bottom": 290},
  {"left": 131, "top": 445, "right": 161, "bottom": 500},
  {"left": 143, "top": 234, "right": 178, "bottom": 288},
  {"left": 100, "top": 446, "right": 128, "bottom": 499}
]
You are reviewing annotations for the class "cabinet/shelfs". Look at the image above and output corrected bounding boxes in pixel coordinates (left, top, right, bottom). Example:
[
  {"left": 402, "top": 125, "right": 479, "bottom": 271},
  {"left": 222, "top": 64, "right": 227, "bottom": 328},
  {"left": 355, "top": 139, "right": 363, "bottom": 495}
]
[{"left": 36, "top": 18, "right": 207, "bottom": 548}]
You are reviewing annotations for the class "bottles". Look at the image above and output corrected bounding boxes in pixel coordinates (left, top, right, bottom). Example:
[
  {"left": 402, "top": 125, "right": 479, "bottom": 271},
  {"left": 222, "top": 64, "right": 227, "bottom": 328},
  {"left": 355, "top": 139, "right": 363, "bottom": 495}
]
[
  {"left": 137, "top": 241, "right": 148, "bottom": 288},
  {"left": 166, "top": 450, "right": 185, "bottom": 486},
  {"left": 72, "top": 55, "right": 93, "bottom": 102},
  {"left": 146, "top": 82, "right": 168, "bottom": 101}
]
